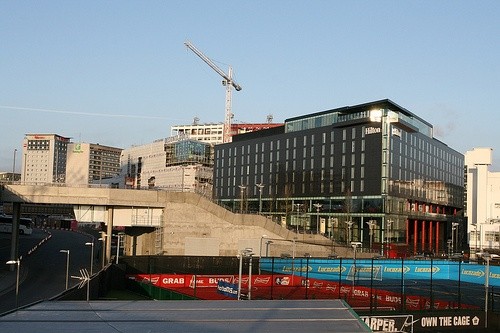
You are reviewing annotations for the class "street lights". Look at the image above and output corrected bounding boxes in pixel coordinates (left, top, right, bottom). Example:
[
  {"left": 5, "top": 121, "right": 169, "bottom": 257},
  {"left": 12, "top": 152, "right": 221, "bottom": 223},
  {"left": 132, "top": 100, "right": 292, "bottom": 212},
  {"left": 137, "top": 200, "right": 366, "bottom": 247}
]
[
  {"left": 293, "top": 203, "right": 303, "bottom": 234},
  {"left": 451, "top": 257, "right": 461, "bottom": 316},
  {"left": 312, "top": 203, "right": 323, "bottom": 234},
  {"left": 393, "top": 256, "right": 404, "bottom": 313},
  {"left": 337, "top": 255, "right": 342, "bottom": 300},
  {"left": 6, "top": 260, "right": 21, "bottom": 305},
  {"left": 238, "top": 185, "right": 246, "bottom": 214},
  {"left": 304, "top": 252, "right": 310, "bottom": 300},
  {"left": 12, "top": 148, "right": 18, "bottom": 184},
  {"left": 426, "top": 253, "right": 433, "bottom": 312},
  {"left": 59, "top": 249, "right": 71, "bottom": 291},
  {"left": 236, "top": 248, "right": 256, "bottom": 300},
  {"left": 366, "top": 220, "right": 375, "bottom": 252},
  {"left": 451, "top": 222, "right": 459, "bottom": 254},
  {"left": 256, "top": 183, "right": 265, "bottom": 212},
  {"left": 330, "top": 218, "right": 336, "bottom": 239},
  {"left": 370, "top": 256, "right": 379, "bottom": 314},
  {"left": 115, "top": 235, "right": 120, "bottom": 265},
  {"left": 84, "top": 242, "right": 94, "bottom": 277},
  {"left": 345, "top": 220, "right": 354, "bottom": 246},
  {"left": 386, "top": 220, "right": 394, "bottom": 257},
  {"left": 98, "top": 237, "right": 105, "bottom": 269}
]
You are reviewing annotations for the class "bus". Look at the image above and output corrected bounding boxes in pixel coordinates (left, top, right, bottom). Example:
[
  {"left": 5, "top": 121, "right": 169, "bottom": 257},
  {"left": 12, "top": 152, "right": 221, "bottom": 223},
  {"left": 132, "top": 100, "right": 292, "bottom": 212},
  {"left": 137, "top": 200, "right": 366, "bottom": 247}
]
[
  {"left": 0, "top": 215, "right": 36, "bottom": 235},
  {"left": 0, "top": 172, "right": 21, "bottom": 181}
]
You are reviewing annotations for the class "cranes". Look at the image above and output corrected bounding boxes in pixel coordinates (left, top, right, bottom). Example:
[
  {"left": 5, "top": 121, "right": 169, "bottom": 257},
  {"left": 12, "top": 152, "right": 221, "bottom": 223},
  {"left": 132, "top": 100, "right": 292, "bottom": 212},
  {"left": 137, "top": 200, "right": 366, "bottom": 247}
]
[{"left": 184, "top": 39, "right": 242, "bottom": 144}]
